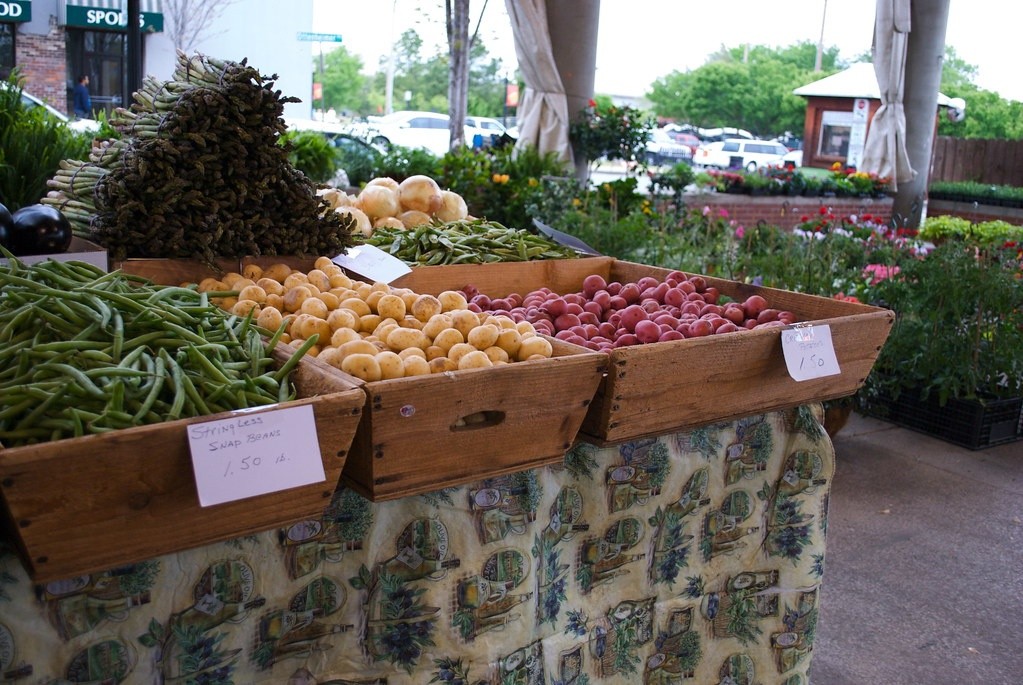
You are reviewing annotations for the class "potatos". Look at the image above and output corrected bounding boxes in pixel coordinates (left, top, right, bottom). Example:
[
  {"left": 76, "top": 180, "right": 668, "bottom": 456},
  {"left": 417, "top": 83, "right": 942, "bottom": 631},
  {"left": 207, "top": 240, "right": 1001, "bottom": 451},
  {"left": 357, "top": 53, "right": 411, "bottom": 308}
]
[
  {"left": 198, "top": 259, "right": 552, "bottom": 383},
  {"left": 456, "top": 271, "right": 796, "bottom": 355}
]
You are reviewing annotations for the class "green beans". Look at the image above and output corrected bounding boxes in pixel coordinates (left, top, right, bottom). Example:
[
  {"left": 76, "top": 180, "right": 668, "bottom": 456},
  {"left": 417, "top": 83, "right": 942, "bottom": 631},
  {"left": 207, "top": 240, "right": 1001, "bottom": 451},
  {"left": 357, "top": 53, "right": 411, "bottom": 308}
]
[
  {"left": 0, "top": 245, "right": 320, "bottom": 448},
  {"left": 354, "top": 211, "right": 585, "bottom": 266}
]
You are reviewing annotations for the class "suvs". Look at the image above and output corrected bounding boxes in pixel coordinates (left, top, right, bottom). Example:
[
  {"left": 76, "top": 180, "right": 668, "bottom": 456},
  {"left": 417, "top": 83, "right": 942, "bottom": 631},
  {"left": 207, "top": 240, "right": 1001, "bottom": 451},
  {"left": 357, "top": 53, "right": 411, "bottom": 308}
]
[{"left": 719, "top": 138, "right": 791, "bottom": 171}]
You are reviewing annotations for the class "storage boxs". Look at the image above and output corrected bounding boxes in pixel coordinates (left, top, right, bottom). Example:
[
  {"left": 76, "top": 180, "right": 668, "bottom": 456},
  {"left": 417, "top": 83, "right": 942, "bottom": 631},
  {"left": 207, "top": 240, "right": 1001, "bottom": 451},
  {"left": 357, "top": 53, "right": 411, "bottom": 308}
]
[
  {"left": 854, "top": 381, "right": 1023, "bottom": 452},
  {"left": 1, "top": 235, "right": 896, "bottom": 584}
]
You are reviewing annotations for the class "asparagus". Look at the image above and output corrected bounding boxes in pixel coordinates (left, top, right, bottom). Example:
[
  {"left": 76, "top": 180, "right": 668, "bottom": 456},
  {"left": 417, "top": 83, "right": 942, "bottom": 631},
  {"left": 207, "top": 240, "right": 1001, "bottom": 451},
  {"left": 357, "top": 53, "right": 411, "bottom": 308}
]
[{"left": 38, "top": 47, "right": 366, "bottom": 274}]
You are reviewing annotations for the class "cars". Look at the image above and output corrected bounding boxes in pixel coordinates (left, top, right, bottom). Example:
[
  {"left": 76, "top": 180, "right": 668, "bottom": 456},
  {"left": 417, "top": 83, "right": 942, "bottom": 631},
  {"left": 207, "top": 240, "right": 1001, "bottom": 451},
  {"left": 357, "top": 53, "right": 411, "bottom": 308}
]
[
  {"left": 641, "top": 126, "right": 692, "bottom": 167},
  {"left": 674, "top": 132, "right": 735, "bottom": 168},
  {"left": 0, "top": 80, "right": 102, "bottom": 139},
  {"left": 369, "top": 110, "right": 519, "bottom": 156},
  {"left": 324, "top": 127, "right": 387, "bottom": 164}
]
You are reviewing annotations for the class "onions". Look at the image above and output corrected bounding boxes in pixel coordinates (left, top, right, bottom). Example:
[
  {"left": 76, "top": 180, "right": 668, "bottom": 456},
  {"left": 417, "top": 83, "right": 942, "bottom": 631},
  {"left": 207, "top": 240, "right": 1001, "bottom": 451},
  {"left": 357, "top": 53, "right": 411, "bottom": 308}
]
[{"left": 313, "top": 176, "right": 469, "bottom": 237}]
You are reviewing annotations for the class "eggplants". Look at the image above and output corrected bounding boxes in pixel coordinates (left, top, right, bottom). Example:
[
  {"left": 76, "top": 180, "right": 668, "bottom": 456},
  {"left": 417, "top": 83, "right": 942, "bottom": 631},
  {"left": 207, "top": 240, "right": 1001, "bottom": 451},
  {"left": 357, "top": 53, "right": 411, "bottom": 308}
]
[{"left": 0, "top": 202, "right": 72, "bottom": 256}]
